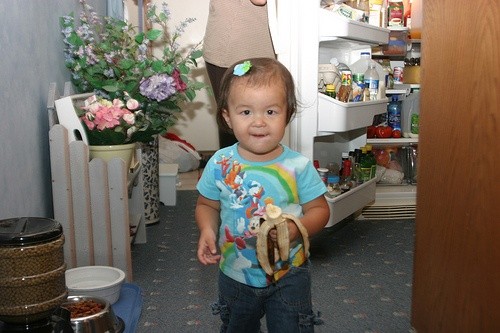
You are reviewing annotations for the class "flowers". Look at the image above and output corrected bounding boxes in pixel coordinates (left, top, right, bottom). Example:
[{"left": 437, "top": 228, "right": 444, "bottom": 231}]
[{"left": 58, "top": 0, "right": 212, "bottom": 145}]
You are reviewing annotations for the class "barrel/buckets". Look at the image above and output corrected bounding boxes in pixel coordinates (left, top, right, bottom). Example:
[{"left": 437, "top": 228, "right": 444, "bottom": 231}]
[{"left": 0, "top": 216, "right": 71, "bottom": 333}]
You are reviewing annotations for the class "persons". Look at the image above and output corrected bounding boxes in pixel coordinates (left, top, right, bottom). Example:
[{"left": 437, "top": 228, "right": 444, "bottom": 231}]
[
  {"left": 203, "top": 0, "right": 279, "bottom": 152},
  {"left": 195, "top": 55, "right": 330, "bottom": 333}
]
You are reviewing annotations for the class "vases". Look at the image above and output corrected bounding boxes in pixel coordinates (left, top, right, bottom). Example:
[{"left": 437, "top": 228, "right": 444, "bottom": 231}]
[
  {"left": 141, "top": 137, "right": 161, "bottom": 226},
  {"left": 90, "top": 142, "right": 138, "bottom": 169}
]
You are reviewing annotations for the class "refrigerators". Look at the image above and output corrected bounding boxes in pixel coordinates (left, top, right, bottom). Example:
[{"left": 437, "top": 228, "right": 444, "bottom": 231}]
[{"left": 266, "top": 0, "right": 422, "bottom": 228}]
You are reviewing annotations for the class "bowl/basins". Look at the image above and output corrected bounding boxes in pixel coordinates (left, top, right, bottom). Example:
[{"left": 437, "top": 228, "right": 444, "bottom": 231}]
[
  {"left": 66, "top": 266, "right": 126, "bottom": 305},
  {"left": 66, "top": 295, "right": 125, "bottom": 333}
]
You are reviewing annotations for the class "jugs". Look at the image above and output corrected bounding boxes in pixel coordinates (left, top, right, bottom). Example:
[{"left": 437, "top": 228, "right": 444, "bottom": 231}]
[{"left": 402, "top": 143, "right": 418, "bottom": 183}]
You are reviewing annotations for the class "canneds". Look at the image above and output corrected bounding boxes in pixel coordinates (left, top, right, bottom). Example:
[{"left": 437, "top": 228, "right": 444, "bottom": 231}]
[{"left": 393, "top": 67, "right": 403, "bottom": 81}]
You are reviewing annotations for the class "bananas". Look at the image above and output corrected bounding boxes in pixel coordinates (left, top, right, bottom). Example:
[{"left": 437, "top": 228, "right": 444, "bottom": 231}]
[{"left": 256, "top": 203, "right": 310, "bottom": 276}]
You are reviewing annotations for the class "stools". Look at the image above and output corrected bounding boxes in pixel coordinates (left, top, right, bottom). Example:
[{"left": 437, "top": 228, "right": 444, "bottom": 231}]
[{"left": 159, "top": 163, "right": 179, "bottom": 208}]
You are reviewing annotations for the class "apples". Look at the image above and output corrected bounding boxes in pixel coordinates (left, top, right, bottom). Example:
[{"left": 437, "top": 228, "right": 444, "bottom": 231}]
[{"left": 367, "top": 125, "right": 401, "bottom": 138}]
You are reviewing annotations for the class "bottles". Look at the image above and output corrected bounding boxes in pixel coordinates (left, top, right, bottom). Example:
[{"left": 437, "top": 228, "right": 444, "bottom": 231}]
[
  {"left": 410, "top": 0, "right": 423, "bottom": 39},
  {"left": 403, "top": 56, "right": 420, "bottom": 66},
  {"left": 319, "top": 51, "right": 394, "bottom": 103},
  {"left": 346, "top": 0, "right": 369, "bottom": 11},
  {"left": 388, "top": 95, "right": 402, "bottom": 131},
  {"left": 316, "top": 143, "right": 377, "bottom": 198}
]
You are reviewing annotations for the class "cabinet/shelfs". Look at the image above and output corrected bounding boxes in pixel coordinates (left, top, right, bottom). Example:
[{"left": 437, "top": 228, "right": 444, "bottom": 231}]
[{"left": 49, "top": 123, "right": 147, "bottom": 284}]
